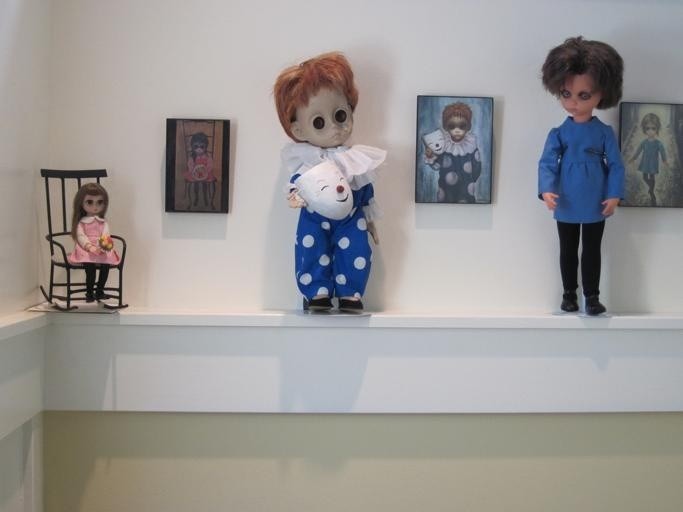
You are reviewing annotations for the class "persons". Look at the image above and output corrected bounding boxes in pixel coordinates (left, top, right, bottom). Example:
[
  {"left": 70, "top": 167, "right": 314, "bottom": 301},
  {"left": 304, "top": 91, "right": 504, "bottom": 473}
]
[
  {"left": 273, "top": 50, "right": 385, "bottom": 314},
  {"left": 423, "top": 101, "right": 479, "bottom": 203},
  {"left": 184, "top": 131, "right": 214, "bottom": 182},
  {"left": 627, "top": 112, "right": 671, "bottom": 205},
  {"left": 538, "top": 34, "right": 625, "bottom": 318},
  {"left": 68, "top": 183, "right": 119, "bottom": 302}
]
